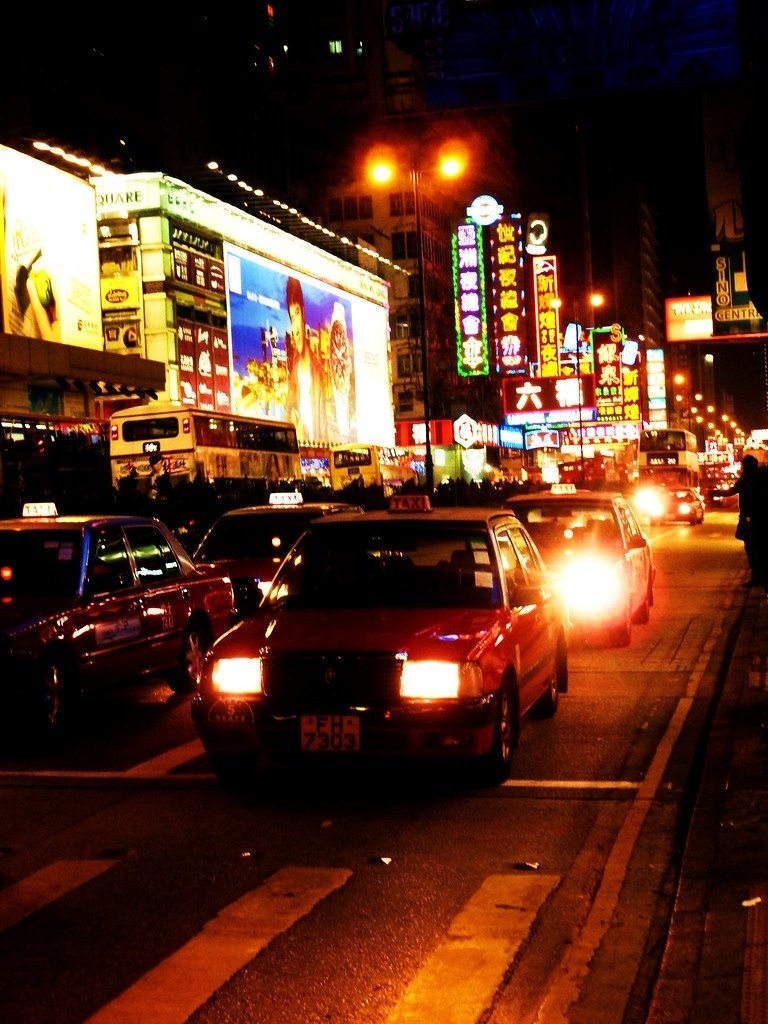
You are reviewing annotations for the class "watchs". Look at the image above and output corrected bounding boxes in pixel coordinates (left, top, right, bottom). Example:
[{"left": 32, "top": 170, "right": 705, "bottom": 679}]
[{"left": 330, "top": 302, "right": 351, "bottom": 435}]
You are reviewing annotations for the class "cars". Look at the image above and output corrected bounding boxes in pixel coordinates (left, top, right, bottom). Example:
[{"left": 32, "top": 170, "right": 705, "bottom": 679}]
[
  {"left": 701, "top": 479, "right": 735, "bottom": 505},
  {"left": 501, "top": 484, "right": 656, "bottom": 647},
  {"left": 189, "top": 494, "right": 567, "bottom": 792},
  {"left": 192, "top": 492, "right": 366, "bottom": 621},
  {"left": 0, "top": 504, "right": 240, "bottom": 737},
  {"left": 649, "top": 484, "right": 706, "bottom": 526}
]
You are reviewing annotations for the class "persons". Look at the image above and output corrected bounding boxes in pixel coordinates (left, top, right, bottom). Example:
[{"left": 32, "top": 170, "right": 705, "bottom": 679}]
[
  {"left": 708, "top": 456, "right": 768, "bottom": 588},
  {"left": 284, "top": 278, "right": 328, "bottom": 441},
  {"left": 146, "top": 455, "right": 171, "bottom": 501}
]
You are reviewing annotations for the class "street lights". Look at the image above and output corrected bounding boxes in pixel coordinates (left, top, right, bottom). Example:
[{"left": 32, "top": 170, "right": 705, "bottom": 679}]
[
  {"left": 547, "top": 295, "right": 606, "bottom": 491},
  {"left": 367, "top": 142, "right": 470, "bottom": 498}
]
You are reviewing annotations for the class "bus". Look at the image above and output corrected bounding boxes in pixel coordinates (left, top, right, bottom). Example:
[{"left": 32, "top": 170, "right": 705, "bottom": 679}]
[
  {"left": 328, "top": 444, "right": 421, "bottom": 492},
  {"left": 557, "top": 461, "right": 605, "bottom": 483},
  {"left": 636, "top": 428, "right": 699, "bottom": 488},
  {"left": 108, "top": 404, "right": 303, "bottom": 490}
]
